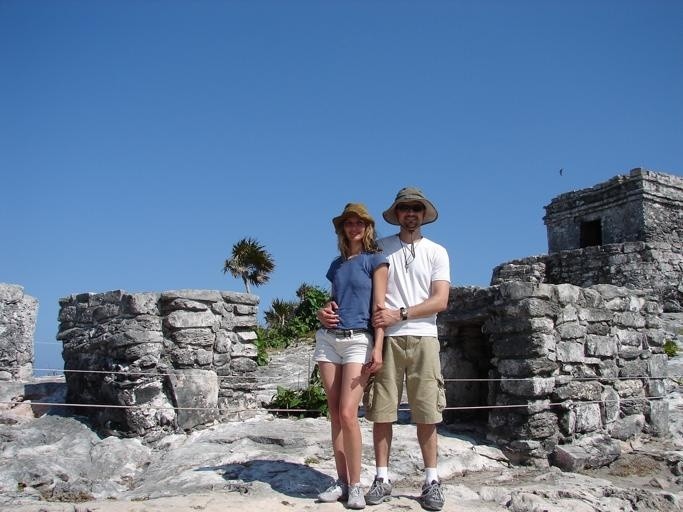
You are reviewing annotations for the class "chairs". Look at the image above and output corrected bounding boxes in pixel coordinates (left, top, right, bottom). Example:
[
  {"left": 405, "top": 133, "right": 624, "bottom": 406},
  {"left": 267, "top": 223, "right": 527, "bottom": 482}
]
[
  {"left": 364, "top": 475, "right": 393, "bottom": 505},
  {"left": 421, "top": 479, "right": 444, "bottom": 511},
  {"left": 347, "top": 482, "right": 366, "bottom": 510},
  {"left": 316, "top": 479, "right": 348, "bottom": 503}
]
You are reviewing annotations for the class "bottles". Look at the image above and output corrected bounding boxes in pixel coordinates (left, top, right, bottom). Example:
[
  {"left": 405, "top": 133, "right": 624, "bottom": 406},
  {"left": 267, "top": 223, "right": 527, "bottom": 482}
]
[
  {"left": 382, "top": 187, "right": 439, "bottom": 225},
  {"left": 332, "top": 202, "right": 374, "bottom": 234}
]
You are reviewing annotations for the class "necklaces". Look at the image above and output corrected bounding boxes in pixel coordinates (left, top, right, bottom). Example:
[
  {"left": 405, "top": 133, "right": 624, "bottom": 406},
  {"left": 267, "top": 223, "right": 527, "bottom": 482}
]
[{"left": 398, "top": 234, "right": 419, "bottom": 273}]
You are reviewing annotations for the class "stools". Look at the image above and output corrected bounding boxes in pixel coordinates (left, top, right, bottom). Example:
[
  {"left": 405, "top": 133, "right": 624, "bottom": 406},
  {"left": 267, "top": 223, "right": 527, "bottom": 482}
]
[{"left": 327, "top": 329, "right": 367, "bottom": 337}]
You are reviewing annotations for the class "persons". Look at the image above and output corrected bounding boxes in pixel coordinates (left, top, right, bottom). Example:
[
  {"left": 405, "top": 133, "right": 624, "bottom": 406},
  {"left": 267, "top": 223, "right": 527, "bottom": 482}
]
[
  {"left": 317, "top": 203, "right": 390, "bottom": 509},
  {"left": 316, "top": 188, "right": 451, "bottom": 510}
]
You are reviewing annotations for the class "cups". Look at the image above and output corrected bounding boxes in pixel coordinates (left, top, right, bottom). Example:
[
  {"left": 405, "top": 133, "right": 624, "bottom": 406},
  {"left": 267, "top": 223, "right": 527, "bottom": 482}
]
[{"left": 395, "top": 203, "right": 425, "bottom": 212}]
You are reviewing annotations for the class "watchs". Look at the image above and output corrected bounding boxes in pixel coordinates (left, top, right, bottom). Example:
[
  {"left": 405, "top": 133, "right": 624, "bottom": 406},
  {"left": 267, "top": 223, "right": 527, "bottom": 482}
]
[{"left": 400, "top": 307, "right": 407, "bottom": 321}]
